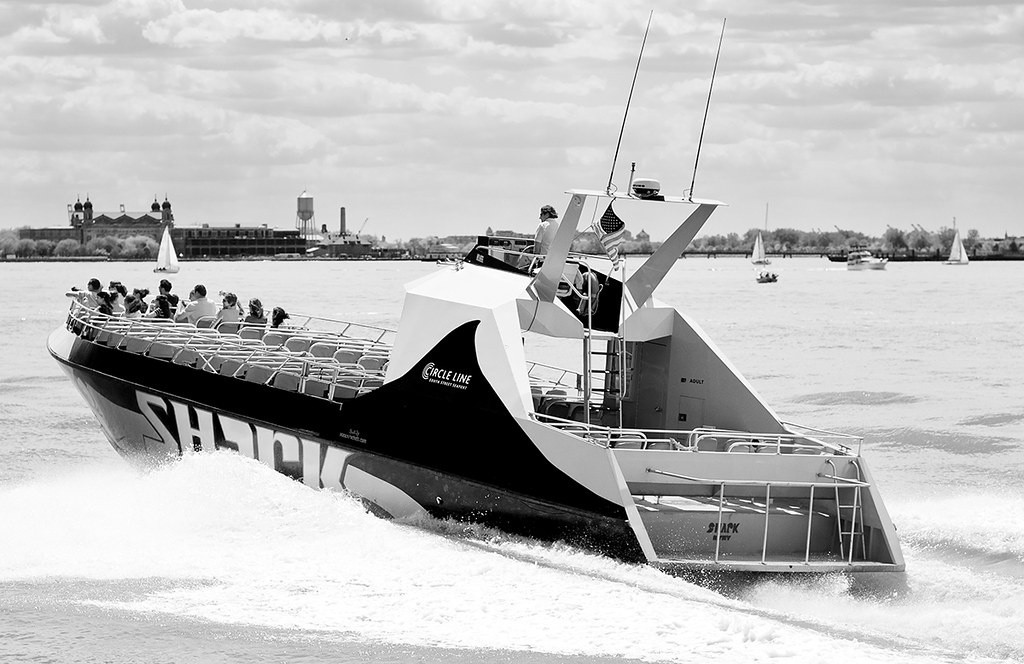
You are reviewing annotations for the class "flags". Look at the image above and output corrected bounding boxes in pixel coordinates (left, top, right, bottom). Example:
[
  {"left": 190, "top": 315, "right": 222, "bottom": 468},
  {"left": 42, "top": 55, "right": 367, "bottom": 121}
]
[{"left": 590, "top": 202, "right": 626, "bottom": 272}]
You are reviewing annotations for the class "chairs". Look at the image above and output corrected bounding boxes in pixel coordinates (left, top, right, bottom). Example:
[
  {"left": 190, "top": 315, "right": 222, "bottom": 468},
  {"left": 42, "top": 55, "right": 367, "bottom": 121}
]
[
  {"left": 531, "top": 382, "right": 824, "bottom": 457},
  {"left": 67, "top": 304, "right": 396, "bottom": 404}
]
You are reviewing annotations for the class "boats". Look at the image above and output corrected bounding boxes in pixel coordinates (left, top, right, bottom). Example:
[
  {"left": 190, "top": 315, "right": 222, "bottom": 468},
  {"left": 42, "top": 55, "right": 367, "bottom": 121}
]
[
  {"left": 825, "top": 248, "right": 849, "bottom": 262},
  {"left": 756, "top": 274, "right": 778, "bottom": 283},
  {"left": 844, "top": 244, "right": 889, "bottom": 270},
  {"left": 45, "top": 8, "right": 911, "bottom": 589}
]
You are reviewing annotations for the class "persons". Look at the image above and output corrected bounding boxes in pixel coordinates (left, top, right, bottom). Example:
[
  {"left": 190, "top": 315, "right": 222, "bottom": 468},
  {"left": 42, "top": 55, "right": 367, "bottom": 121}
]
[
  {"left": 66, "top": 279, "right": 216, "bottom": 324},
  {"left": 271, "top": 307, "right": 290, "bottom": 331},
  {"left": 209, "top": 293, "right": 242, "bottom": 334},
  {"left": 93, "top": 291, "right": 113, "bottom": 317},
  {"left": 241, "top": 298, "right": 267, "bottom": 333},
  {"left": 119, "top": 295, "right": 142, "bottom": 323},
  {"left": 532, "top": 205, "right": 561, "bottom": 268},
  {"left": 144, "top": 294, "right": 173, "bottom": 319}
]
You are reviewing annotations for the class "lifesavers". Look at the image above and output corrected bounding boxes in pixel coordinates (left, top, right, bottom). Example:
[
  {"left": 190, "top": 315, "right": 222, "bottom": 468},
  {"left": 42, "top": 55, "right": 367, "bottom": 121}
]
[{"left": 574, "top": 272, "right": 600, "bottom": 316}]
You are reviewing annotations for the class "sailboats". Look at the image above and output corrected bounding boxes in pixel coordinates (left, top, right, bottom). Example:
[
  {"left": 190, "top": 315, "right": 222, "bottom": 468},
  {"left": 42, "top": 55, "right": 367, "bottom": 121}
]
[
  {"left": 940, "top": 231, "right": 970, "bottom": 265},
  {"left": 152, "top": 224, "right": 182, "bottom": 274},
  {"left": 750, "top": 231, "right": 772, "bottom": 265}
]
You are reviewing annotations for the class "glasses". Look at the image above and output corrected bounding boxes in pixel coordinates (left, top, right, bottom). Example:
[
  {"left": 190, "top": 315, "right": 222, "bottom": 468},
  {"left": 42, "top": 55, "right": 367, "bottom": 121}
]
[
  {"left": 540, "top": 212, "right": 543, "bottom": 216},
  {"left": 223, "top": 299, "right": 227, "bottom": 302}
]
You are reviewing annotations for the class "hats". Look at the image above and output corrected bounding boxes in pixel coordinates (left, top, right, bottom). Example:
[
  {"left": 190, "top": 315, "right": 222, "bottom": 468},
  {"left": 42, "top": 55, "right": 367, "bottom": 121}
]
[{"left": 541, "top": 205, "right": 559, "bottom": 217}]
